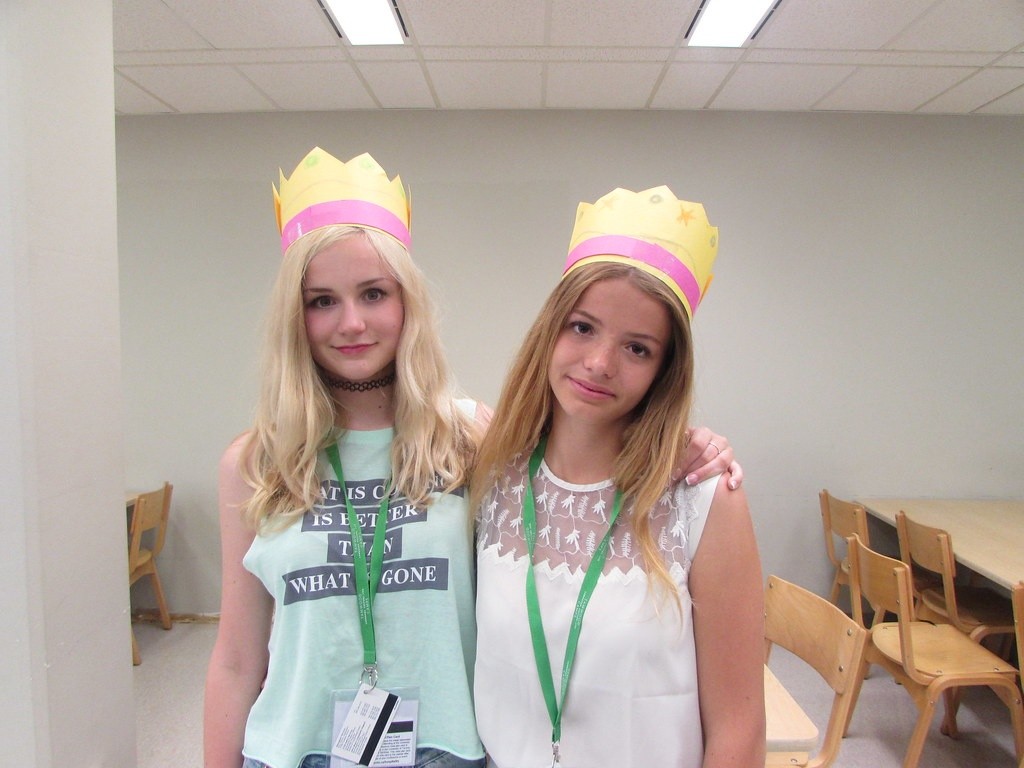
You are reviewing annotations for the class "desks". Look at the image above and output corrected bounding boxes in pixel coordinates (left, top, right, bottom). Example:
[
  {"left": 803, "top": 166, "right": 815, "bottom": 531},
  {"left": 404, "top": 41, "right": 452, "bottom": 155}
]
[{"left": 853, "top": 496, "right": 1024, "bottom": 592}]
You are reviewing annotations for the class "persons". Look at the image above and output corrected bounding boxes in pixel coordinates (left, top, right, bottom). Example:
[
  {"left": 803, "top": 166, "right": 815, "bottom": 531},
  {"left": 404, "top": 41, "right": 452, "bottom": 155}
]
[
  {"left": 472, "top": 184, "right": 767, "bottom": 768},
  {"left": 202, "top": 146, "right": 745, "bottom": 768}
]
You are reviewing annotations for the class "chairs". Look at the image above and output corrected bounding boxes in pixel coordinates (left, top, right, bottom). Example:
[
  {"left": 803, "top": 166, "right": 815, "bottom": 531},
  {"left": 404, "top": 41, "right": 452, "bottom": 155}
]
[
  {"left": 129, "top": 480, "right": 174, "bottom": 666},
  {"left": 764, "top": 489, "right": 1024, "bottom": 768}
]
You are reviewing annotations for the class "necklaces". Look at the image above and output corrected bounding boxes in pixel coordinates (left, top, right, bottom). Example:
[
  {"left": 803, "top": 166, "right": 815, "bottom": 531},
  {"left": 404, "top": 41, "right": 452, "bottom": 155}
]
[{"left": 324, "top": 373, "right": 395, "bottom": 392}]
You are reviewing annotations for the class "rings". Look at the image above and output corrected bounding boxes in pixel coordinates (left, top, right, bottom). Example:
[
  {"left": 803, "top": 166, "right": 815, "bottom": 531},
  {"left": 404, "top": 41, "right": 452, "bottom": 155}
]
[{"left": 709, "top": 442, "right": 721, "bottom": 453}]
